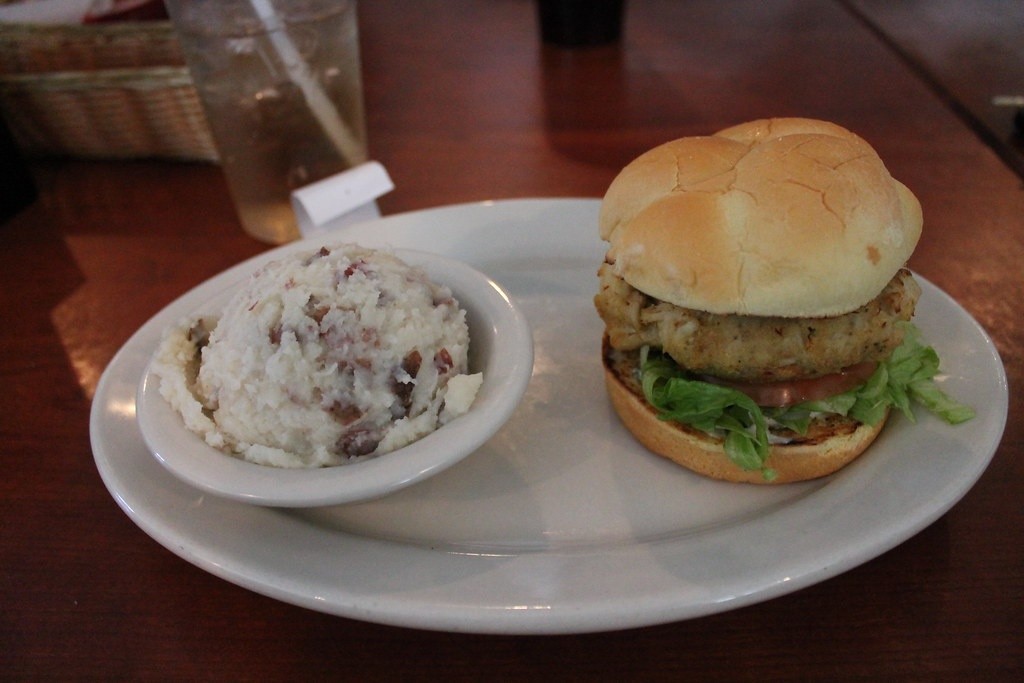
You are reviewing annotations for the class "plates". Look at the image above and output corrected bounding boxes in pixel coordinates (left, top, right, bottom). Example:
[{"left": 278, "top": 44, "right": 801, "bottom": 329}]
[{"left": 90, "top": 199, "right": 1011, "bottom": 638}]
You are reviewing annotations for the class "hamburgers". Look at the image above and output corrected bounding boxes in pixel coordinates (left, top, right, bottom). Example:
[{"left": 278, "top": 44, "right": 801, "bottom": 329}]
[{"left": 594, "top": 118, "right": 975, "bottom": 486}]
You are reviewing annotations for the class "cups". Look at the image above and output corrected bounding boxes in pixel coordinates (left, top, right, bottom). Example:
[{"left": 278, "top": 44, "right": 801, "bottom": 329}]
[{"left": 164, "top": 1, "right": 375, "bottom": 247}]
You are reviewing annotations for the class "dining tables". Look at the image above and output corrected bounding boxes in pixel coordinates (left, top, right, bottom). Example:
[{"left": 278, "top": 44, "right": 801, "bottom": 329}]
[{"left": 0, "top": 0, "right": 1024, "bottom": 683}]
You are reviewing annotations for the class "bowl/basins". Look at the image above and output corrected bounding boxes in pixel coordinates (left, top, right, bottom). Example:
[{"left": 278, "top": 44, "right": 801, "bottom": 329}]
[{"left": 135, "top": 247, "right": 532, "bottom": 506}]
